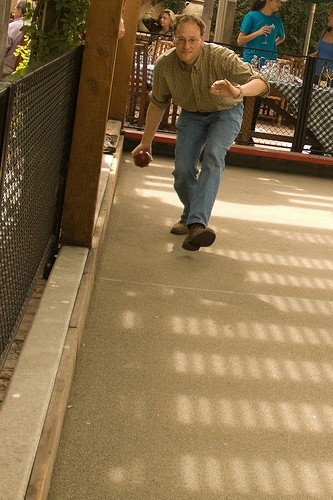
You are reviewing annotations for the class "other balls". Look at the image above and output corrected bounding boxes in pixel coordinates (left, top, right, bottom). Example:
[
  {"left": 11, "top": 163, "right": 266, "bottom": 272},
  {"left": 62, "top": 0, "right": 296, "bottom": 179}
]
[{"left": 134, "top": 151, "right": 152, "bottom": 167}]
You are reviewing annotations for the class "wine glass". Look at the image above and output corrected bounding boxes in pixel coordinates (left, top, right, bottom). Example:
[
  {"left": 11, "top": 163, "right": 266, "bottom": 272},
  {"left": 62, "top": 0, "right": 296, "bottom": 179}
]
[
  {"left": 325, "top": 64, "right": 333, "bottom": 89},
  {"left": 261, "top": 24, "right": 271, "bottom": 45}
]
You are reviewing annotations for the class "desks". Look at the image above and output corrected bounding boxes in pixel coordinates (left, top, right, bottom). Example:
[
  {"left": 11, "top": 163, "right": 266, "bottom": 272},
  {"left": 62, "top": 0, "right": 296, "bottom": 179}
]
[{"left": 268, "top": 80, "right": 333, "bottom": 156}]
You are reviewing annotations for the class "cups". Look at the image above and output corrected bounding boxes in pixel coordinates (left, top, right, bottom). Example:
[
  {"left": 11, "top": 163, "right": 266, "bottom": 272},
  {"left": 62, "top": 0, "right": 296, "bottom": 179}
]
[{"left": 258, "top": 58, "right": 268, "bottom": 69}]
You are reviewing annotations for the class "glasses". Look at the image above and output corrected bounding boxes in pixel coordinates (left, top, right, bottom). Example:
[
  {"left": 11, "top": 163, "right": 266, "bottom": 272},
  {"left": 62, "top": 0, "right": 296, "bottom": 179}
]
[{"left": 173, "top": 36, "right": 201, "bottom": 45}]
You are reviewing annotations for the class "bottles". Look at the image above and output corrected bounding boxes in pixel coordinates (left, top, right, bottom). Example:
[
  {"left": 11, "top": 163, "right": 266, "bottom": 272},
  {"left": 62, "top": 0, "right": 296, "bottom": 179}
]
[{"left": 318, "top": 62, "right": 330, "bottom": 88}]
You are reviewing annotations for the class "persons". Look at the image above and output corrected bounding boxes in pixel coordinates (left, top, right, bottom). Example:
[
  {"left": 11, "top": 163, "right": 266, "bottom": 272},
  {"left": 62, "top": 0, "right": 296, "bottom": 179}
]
[
  {"left": 3, "top": 0, "right": 37, "bottom": 77},
  {"left": 237, "top": 0, "right": 285, "bottom": 147},
  {"left": 132, "top": 15, "right": 270, "bottom": 252},
  {"left": 142, "top": 9, "right": 176, "bottom": 45},
  {"left": 310, "top": 11, "right": 333, "bottom": 155}
]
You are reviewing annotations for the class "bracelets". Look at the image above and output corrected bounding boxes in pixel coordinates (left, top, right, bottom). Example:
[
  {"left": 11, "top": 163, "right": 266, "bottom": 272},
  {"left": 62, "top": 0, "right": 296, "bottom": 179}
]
[{"left": 233, "top": 84, "right": 243, "bottom": 100}]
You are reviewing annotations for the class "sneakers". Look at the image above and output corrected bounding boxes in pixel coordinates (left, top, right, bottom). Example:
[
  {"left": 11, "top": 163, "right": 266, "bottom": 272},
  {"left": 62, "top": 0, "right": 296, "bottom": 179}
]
[
  {"left": 171, "top": 220, "right": 187, "bottom": 235},
  {"left": 182, "top": 226, "right": 216, "bottom": 251}
]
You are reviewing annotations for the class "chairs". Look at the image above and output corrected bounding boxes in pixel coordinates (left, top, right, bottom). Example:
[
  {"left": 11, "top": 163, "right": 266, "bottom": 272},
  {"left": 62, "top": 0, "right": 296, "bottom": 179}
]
[
  {"left": 259, "top": 58, "right": 295, "bottom": 128},
  {"left": 125, "top": 32, "right": 180, "bottom": 129}
]
